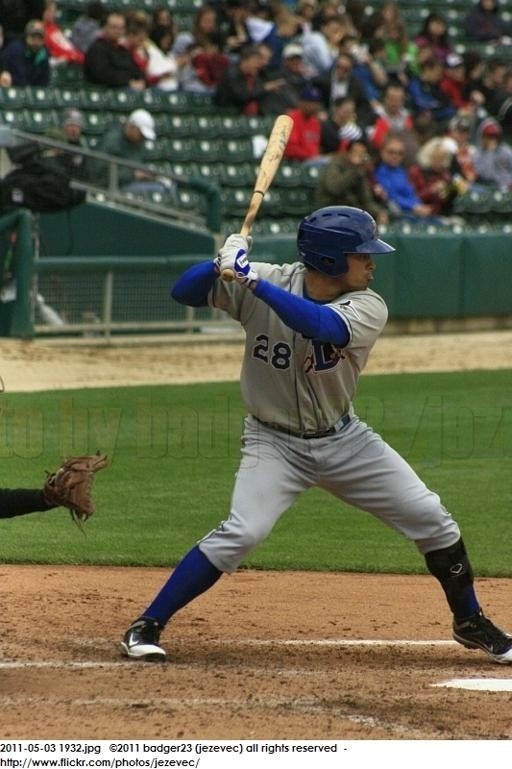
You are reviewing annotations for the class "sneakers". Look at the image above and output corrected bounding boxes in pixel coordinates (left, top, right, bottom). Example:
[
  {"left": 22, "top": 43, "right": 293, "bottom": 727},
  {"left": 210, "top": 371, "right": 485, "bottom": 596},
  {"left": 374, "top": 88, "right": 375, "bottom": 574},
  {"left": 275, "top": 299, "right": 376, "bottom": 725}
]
[
  {"left": 452, "top": 607, "right": 512, "bottom": 664},
  {"left": 120, "top": 617, "right": 168, "bottom": 662}
]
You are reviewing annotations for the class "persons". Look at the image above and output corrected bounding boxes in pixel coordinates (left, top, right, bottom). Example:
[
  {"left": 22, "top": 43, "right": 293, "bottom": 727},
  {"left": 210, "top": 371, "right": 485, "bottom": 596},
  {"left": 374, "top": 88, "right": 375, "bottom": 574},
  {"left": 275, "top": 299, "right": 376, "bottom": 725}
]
[
  {"left": 1, "top": 0, "right": 512, "bottom": 221},
  {"left": 0, "top": 446, "right": 110, "bottom": 520},
  {"left": 119, "top": 206, "right": 512, "bottom": 667}
]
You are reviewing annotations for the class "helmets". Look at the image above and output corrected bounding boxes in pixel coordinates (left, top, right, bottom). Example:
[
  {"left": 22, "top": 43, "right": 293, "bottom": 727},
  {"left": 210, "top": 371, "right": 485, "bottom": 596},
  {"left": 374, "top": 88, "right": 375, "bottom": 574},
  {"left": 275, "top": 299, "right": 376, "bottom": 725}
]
[{"left": 297, "top": 206, "right": 395, "bottom": 277}]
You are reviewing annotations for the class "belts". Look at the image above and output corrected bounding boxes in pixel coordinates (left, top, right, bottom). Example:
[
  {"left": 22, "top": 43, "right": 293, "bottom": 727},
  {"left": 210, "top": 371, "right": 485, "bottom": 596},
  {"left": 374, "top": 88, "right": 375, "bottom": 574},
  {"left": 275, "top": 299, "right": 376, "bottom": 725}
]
[{"left": 248, "top": 415, "right": 350, "bottom": 438}]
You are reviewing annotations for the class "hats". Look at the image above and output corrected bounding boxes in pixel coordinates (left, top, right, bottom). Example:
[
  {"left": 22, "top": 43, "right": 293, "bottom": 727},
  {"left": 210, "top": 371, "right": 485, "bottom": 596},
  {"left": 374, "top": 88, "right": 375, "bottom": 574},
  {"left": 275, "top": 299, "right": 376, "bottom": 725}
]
[
  {"left": 283, "top": 45, "right": 303, "bottom": 59},
  {"left": 130, "top": 109, "right": 157, "bottom": 140},
  {"left": 26, "top": 20, "right": 46, "bottom": 37}
]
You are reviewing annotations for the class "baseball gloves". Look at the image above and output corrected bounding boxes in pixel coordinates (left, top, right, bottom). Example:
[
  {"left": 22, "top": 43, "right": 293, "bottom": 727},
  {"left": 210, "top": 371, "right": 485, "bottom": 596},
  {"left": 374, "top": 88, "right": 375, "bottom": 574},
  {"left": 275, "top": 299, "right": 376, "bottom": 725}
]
[{"left": 45, "top": 452, "right": 110, "bottom": 517}]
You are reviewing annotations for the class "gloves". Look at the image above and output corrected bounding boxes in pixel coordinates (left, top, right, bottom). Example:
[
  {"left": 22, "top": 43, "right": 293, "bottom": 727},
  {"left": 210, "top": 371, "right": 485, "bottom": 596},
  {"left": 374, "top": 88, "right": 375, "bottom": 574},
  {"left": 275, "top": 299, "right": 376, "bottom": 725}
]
[{"left": 212, "top": 234, "right": 259, "bottom": 288}]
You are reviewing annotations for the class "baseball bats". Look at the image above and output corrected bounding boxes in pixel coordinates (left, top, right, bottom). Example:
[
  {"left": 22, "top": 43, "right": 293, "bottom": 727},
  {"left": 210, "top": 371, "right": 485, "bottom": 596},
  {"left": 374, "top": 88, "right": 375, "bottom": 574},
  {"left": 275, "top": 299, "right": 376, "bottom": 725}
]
[{"left": 219, "top": 116, "right": 296, "bottom": 283}]
